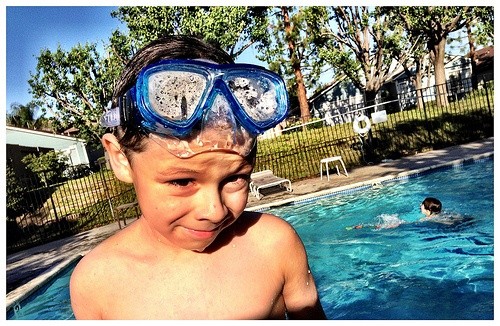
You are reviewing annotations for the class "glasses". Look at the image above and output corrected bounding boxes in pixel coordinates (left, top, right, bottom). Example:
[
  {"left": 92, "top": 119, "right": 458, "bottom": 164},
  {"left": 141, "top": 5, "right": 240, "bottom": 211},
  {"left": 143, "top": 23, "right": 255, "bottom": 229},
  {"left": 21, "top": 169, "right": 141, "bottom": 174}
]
[{"left": 100, "top": 59, "right": 289, "bottom": 158}]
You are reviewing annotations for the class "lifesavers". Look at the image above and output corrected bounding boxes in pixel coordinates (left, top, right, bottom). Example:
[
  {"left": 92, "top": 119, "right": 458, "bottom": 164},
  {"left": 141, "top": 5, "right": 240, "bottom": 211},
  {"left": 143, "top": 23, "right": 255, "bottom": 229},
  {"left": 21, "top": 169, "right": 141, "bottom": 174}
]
[{"left": 353, "top": 115, "right": 371, "bottom": 133}]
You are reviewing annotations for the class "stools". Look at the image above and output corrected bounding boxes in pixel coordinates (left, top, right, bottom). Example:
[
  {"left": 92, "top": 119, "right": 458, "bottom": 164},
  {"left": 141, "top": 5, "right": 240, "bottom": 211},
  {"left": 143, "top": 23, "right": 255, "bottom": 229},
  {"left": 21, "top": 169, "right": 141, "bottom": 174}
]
[{"left": 117, "top": 202, "right": 140, "bottom": 229}]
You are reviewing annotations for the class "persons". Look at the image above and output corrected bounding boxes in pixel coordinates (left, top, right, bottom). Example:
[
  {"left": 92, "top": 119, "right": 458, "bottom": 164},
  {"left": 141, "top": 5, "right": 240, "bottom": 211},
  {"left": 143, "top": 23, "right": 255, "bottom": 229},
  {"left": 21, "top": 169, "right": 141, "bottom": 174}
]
[
  {"left": 414, "top": 197, "right": 462, "bottom": 226},
  {"left": 68, "top": 35, "right": 329, "bottom": 321}
]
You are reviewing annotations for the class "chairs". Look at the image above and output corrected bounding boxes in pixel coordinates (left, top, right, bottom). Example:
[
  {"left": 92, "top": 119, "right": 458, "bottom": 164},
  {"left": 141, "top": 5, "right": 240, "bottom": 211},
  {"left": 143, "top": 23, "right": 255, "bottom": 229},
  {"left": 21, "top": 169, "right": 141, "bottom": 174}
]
[{"left": 249, "top": 169, "right": 292, "bottom": 200}]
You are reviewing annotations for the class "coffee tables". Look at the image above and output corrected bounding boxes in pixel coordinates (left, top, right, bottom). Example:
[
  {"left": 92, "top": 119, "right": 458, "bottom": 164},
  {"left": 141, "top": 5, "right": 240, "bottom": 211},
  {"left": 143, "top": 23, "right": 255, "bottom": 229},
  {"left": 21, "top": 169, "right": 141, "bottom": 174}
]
[{"left": 320, "top": 156, "right": 348, "bottom": 181}]
